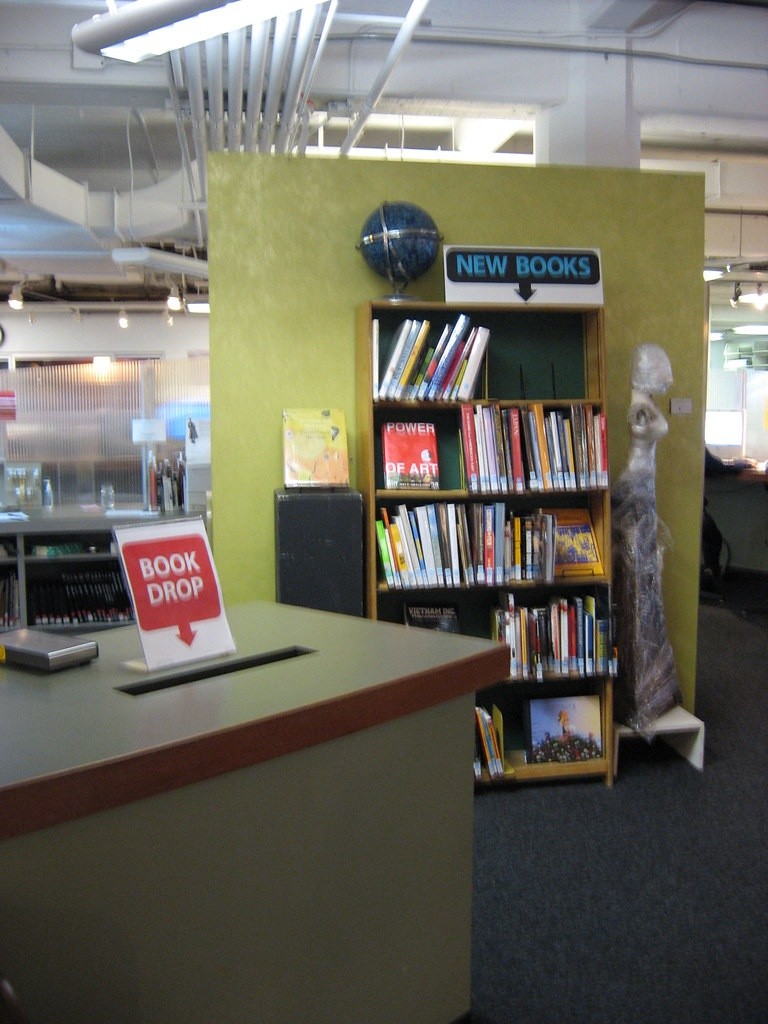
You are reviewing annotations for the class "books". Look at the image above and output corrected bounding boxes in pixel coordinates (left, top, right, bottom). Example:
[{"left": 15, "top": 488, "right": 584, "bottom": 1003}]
[
  {"left": 524, "top": 694, "right": 602, "bottom": 764},
  {"left": 474, "top": 703, "right": 505, "bottom": 778},
  {"left": 381, "top": 402, "right": 609, "bottom": 495},
  {"left": 373, "top": 500, "right": 604, "bottom": 588},
  {"left": 402, "top": 592, "right": 608, "bottom": 684},
  {"left": 282, "top": 407, "right": 350, "bottom": 489},
  {"left": 1, "top": 534, "right": 136, "bottom": 625},
  {"left": 372, "top": 311, "right": 491, "bottom": 402}
]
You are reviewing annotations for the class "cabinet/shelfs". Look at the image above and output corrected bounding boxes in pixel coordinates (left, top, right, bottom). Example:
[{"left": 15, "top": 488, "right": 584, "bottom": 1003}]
[
  {"left": 0, "top": 515, "right": 186, "bottom": 635},
  {"left": 354, "top": 299, "right": 615, "bottom": 790}
]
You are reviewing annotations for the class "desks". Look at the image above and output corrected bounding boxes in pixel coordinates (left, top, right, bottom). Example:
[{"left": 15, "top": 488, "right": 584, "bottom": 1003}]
[{"left": 1, "top": 599, "right": 511, "bottom": 1024}]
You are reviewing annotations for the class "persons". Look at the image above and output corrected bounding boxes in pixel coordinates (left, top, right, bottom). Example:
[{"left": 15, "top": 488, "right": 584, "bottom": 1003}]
[
  {"left": 614, "top": 339, "right": 683, "bottom": 729},
  {"left": 704, "top": 445, "right": 757, "bottom": 585}
]
